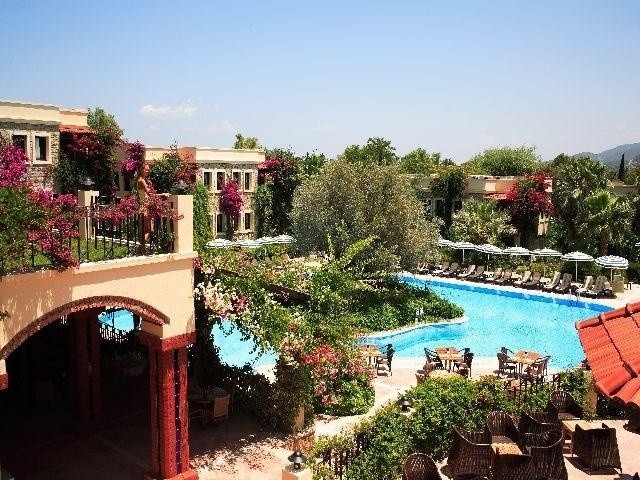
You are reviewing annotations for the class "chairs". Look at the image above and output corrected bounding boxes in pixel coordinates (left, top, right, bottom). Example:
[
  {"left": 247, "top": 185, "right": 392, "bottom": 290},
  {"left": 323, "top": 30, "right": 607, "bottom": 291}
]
[
  {"left": 498, "top": 347, "right": 551, "bottom": 384},
  {"left": 409, "top": 263, "right": 619, "bottom": 297},
  {"left": 187, "top": 377, "right": 237, "bottom": 430},
  {"left": 357, "top": 344, "right": 395, "bottom": 375},
  {"left": 403, "top": 390, "right": 622, "bottom": 480},
  {"left": 416, "top": 347, "right": 474, "bottom": 383}
]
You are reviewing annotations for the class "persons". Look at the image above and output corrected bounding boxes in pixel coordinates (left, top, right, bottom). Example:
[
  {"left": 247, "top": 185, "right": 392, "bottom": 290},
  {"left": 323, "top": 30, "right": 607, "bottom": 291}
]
[{"left": 134, "top": 162, "right": 171, "bottom": 255}]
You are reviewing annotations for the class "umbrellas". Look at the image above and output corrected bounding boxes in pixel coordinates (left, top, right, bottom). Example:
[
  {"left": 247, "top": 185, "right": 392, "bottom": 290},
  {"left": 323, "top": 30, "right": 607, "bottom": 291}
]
[
  {"left": 475, "top": 244, "right": 502, "bottom": 271},
  {"left": 593, "top": 253, "right": 629, "bottom": 287},
  {"left": 269, "top": 234, "right": 298, "bottom": 246},
  {"left": 437, "top": 239, "right": 456, "bottom": 250},
  {"left": 530, "top": 247, "right": 562, "bottom": 278},
  {"left": 228, "top": 240, "right": 244, "bottom": 248},
  {"left": 501, "top": 243, "right": 530, "bottom": 275},
  {"left": 255, "top": 235, "right": 277, "bottom": 246},
  {"left": 237, "top": 239, "right": 262, "bottom": 248},
  {"left": 204, "top": 238, "right": 232, "bottom": 248},
  {"left": 560, "top": 250, "right": 595, "bottom": 283},
  {"left": 449, "top": 241, "right": 477, "bottom": 267}
]
